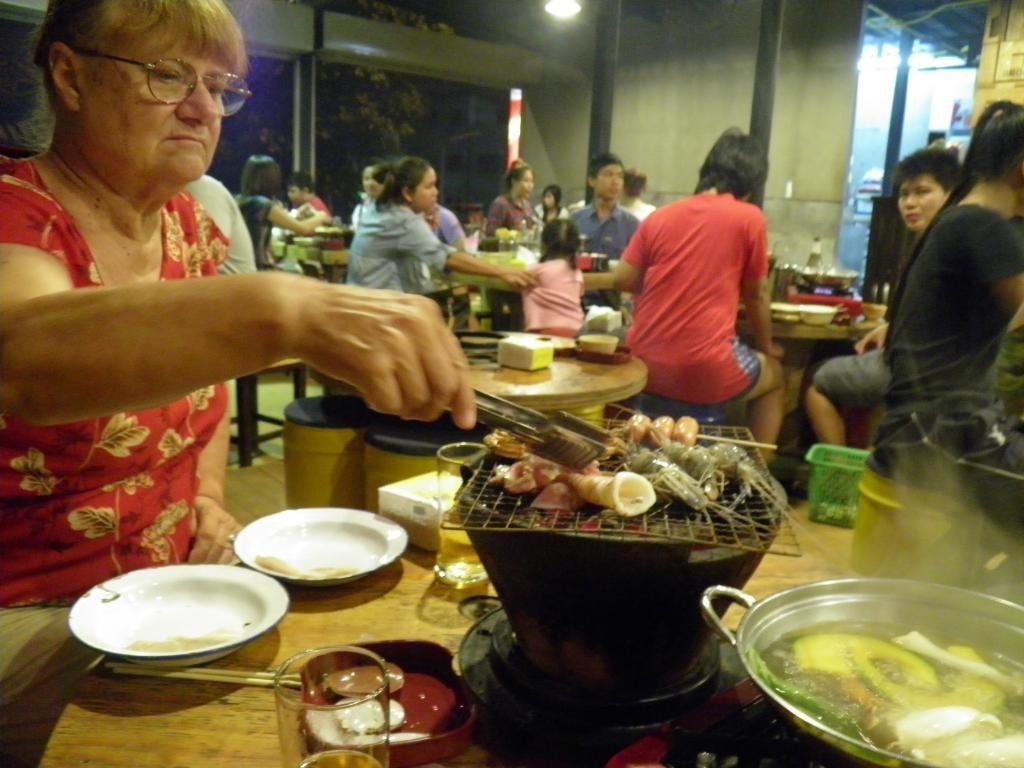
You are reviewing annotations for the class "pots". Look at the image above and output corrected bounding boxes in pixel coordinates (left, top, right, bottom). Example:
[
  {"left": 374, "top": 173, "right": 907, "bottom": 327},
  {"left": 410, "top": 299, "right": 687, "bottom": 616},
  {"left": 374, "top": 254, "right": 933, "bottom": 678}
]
[
  {"left": 799, "top": 266, "right": 859, "bottom": 288},
  {"left": 699, "top": 577, "right": 1024, "bottom": 768}
]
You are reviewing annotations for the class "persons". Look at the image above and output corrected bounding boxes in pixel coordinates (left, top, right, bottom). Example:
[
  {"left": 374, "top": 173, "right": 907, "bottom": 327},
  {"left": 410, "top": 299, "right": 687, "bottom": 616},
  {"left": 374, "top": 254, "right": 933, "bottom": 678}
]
[
  {"left": 615, "top": 125, "right": 785, "bottom": 470},
  {"left": 484, "top": 158, "right": 539, "bottom": 239},
  {"left": 350, "top": 165, "right": 384, "bottom": 232},
  {"left": 520, "top": 218, "right": 586, "bottom": 339},
  {"left": 346, "top": 156, "right": 539, "bottom": 297},
  {"left": 773, "top": 146, "right": 962, "bottom": 499},
  {"left": 275, "top": 169, "right": 331, "bottom": 219},
  {"left": 884, "top": 98, "right": 1024, "bottom": 535},
  {"left": 1, "top": 1, "right": 477, "bottom": 767},
  {"left": 567, "top": 151, "right": 641, "bottom": 344},
  {"left": 423, "top": 198, "right": 466, "bottom": 254},
  {"left": 231, "top": 154, "right": 326, "bottom": 272},
  {"left": 533, "top": 183, "right": 568, "bottom": 228}
]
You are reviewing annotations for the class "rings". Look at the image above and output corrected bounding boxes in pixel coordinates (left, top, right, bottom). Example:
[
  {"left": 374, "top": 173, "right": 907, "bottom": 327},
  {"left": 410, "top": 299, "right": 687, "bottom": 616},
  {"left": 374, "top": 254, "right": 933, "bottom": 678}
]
[{"left": 227, "top": 534, "right": 235, "bottom": 542}]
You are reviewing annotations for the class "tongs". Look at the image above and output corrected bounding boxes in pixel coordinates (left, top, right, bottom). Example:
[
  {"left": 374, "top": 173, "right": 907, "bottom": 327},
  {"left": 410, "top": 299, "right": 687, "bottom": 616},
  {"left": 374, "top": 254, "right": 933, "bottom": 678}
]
[{"left": 444, "top": 382, "right": 614, "bottom": 468}]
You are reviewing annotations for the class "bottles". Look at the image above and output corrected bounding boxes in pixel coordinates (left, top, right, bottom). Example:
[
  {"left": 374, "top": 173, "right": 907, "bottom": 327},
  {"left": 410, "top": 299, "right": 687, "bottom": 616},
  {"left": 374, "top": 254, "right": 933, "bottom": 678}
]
[{"left": 806, "top": 235, "right": 824, "bottom": 267}]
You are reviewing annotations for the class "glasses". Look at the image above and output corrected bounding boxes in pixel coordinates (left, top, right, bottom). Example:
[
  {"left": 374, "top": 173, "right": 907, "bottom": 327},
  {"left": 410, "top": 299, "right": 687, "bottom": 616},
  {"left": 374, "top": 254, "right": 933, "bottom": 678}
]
[{"left": 67, "top": 45, "right": 252, "bottom": 117}]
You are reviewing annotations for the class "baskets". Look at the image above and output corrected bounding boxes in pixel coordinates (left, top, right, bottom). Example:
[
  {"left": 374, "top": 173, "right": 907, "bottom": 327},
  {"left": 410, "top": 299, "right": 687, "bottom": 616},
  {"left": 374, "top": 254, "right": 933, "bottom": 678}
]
[{"left": 805, "top": 443, "right": 871, "bottom": 529}]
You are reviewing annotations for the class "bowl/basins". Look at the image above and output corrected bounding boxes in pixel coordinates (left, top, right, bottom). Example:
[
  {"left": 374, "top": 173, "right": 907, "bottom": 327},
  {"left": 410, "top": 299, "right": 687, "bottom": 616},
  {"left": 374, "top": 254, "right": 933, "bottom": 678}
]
[
  {"left": 767, "top": 300, "right": 803, "bottom": 323},
  {"left": 861, "top": 303, "right": 888, "bottom": 320},
  {"left": 800, "top": 303, "right": 838, "bottom": 327}
]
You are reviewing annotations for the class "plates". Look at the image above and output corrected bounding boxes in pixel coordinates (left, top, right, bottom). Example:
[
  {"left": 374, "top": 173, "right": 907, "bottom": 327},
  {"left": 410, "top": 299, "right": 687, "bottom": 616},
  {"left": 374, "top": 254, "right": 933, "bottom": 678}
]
[
  {"left": 233, "top": 506, "right": 410, "bottom": 585},
  {"left": 68, "top": 564, "right": 292, "bottom": 667}
]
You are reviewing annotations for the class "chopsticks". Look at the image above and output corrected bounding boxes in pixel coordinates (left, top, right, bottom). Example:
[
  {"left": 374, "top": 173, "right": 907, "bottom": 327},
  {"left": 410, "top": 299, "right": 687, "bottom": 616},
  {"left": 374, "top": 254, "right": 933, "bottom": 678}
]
[{"left": 105, "top": 659, "right": 302, "bottom": 692}]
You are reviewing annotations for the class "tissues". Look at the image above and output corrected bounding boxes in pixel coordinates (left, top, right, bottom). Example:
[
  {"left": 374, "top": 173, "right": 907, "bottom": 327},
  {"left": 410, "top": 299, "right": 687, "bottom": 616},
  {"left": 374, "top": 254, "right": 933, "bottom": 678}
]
[
  {"left": 506, "top": 244, "right": 537, "bottom": 273},
  {"left": 582, "top": 304, "right": 626, "bottom": 333},
  {"left": 374, "top": 467, "right": 468, "bottom": 552},
  {"left": 497, "top": 334, "right": 554, "bottom": 372}
]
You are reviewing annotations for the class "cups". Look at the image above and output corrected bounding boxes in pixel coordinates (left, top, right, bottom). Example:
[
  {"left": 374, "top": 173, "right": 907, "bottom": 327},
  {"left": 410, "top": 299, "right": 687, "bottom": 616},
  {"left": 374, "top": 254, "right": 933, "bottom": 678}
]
[
  {"left": 437, "top": 441, "right": 492, "bottom": 590},
  {"left": 273, "top": 644, "right": 391, "bottom": 768}
]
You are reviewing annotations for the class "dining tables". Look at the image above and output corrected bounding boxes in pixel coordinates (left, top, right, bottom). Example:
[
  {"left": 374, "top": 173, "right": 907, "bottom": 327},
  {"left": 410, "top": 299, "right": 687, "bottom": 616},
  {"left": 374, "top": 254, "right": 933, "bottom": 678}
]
[
  {"left": 271, "top": 237, "right": 350, "bottom": 287},
  {"left": 734, "top": 315, "right": 897, "bottom": 470},
  {"left": 44, "top": 497, "right": 866, "bottom": 767},
  {"left": 443, "top": 256, "right": 629, "bottom": 347}
]
